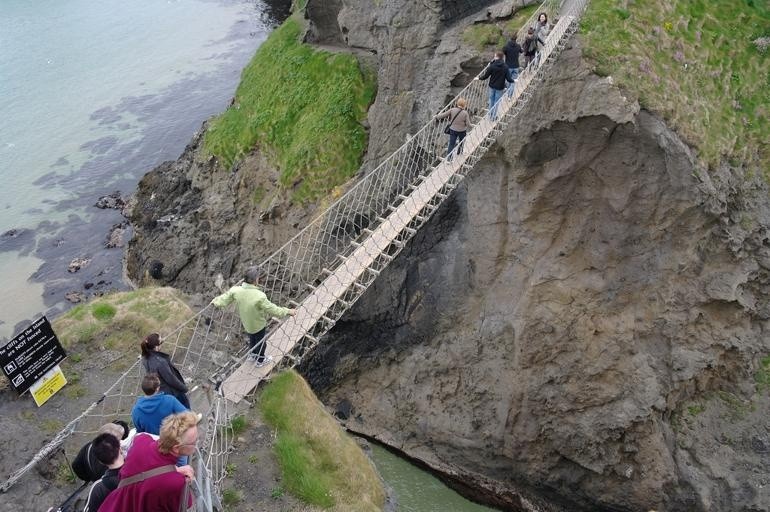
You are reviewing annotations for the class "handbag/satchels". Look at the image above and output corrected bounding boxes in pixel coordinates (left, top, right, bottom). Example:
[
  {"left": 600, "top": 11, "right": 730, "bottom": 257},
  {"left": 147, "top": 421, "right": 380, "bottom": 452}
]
[{"left": 444, "top": 124, "right": 451, "bottom": 134}]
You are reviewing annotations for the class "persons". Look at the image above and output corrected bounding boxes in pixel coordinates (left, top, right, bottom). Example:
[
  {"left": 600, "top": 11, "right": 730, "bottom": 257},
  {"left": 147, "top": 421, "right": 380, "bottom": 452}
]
[
  {"left": 211, "top": 268, "right": 298, "bottom": 368},
  {"left": 436, "top": 98, "right": 474, "bottom": 164},
  {"left": 74, "top": 411, "right": 202, "bottom": 511},
  {"left": 533, "top": 13, "right": 550, "bottom": 63},
  {"left": 475, "top": 51, "right": 518, "bottom": 121},
  {"left": 502, "top": 33, "right": 524, "bottom": 101},
  {"left": 137, "top": 332, "right": 203, "bottom": 425},
  {"left": 131, "top": 374, "right": 190, "bottom": 466},
  {"left": 521, "top": 27, "right": 546, "bottom": 70}
]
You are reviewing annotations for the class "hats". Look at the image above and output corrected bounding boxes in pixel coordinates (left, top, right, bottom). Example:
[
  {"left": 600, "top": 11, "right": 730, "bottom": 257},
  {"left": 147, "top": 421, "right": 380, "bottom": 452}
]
[{"left": 98, "top": 423, "right": 125, "bottom": 442}]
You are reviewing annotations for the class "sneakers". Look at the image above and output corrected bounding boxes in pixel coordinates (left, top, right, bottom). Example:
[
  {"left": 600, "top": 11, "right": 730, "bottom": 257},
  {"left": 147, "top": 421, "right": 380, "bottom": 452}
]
[
  {"left": 197, "top": 413, "right": 203, "bottom": 424},
  {"left": 447, "top": 160, "right": 454, "bottom": 164},
  {"left": 489, "top": 113, "right": 498, "bottom": 122},
  {"left": 247, "top": 354, "right": 272, "bottom": 367}
]
